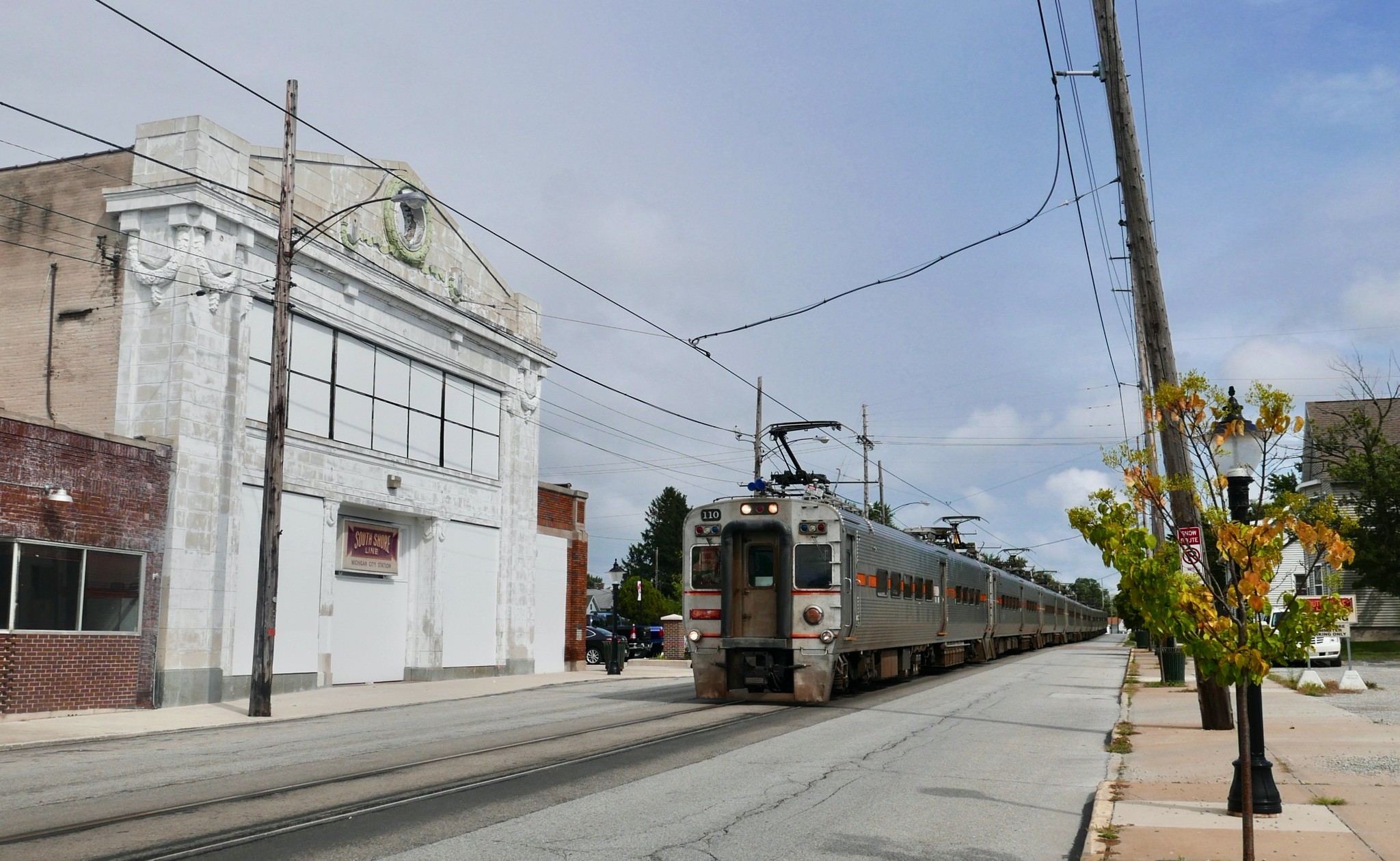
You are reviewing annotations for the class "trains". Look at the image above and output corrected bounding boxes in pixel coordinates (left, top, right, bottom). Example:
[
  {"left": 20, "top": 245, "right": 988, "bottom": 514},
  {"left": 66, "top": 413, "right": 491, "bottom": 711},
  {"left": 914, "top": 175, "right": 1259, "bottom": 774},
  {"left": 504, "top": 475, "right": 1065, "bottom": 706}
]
[{"left": 683, "top": 485, "right": 1109, "bottom": 704}]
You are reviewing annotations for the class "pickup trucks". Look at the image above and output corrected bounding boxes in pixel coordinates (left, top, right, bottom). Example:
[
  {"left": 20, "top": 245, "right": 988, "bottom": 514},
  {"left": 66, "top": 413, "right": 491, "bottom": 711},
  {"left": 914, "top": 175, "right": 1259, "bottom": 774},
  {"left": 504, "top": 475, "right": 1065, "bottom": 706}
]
[
  {"left": 596, "top": 613, "right": 664, "bottom": 644},
  {"left": 586, "top": 613, "right": 652, "bottom": 658}
]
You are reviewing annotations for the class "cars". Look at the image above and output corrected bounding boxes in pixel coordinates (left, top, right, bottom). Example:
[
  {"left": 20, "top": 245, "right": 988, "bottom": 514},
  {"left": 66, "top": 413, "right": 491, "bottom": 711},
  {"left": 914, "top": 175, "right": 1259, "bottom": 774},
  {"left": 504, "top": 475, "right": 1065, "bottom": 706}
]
[
  {"left": 1260, "top": 607, "right": 1342, "bottom": 667},
  {"left": 585, "top": 625, "right": 630, "bottom": 667}
]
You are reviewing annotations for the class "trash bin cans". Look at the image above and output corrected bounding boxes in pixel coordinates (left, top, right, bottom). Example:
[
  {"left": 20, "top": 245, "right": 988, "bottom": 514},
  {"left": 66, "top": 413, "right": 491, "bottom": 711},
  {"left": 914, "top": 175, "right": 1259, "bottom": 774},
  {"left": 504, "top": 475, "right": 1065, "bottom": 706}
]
[
  {"left": 603, "top": 640, "right": 627, "bottom": 671},
  {"left": 1156, "top": 646, "right": 1185, "bottom": 684},
  {"left": 1135, "top": 630, "right": 1149, "bottom": 649}
]
